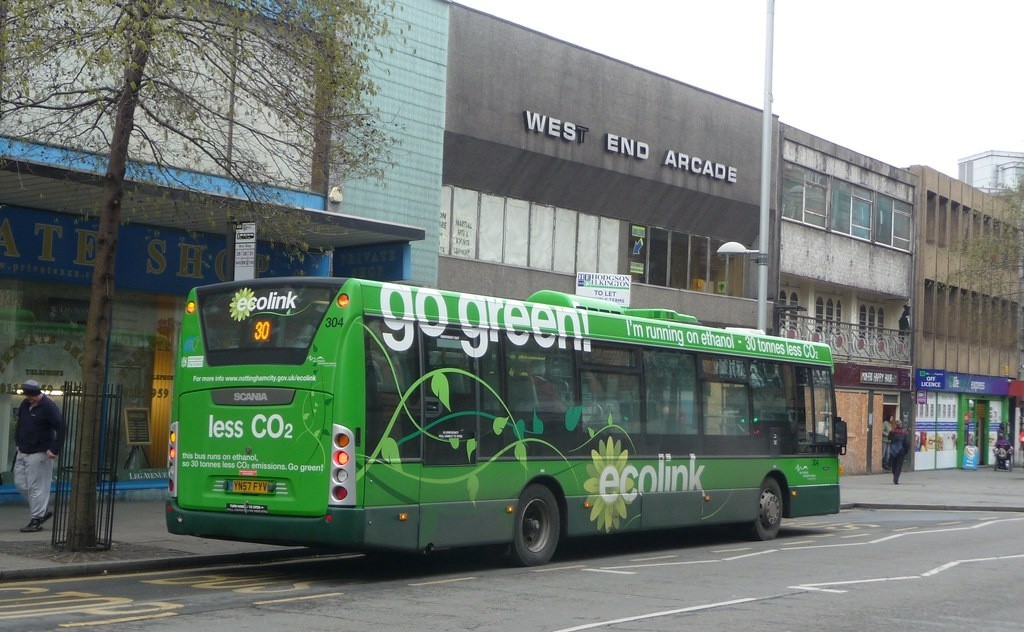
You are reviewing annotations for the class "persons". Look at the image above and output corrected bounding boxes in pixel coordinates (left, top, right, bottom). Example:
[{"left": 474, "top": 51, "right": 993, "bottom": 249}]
[
  {"left": 478, "top": 354, "right": 696, "bottom": 435},
  {"left": 993, "top": 422, "right": 1014, "bottom": 473},
  {"left": 11, "top": 379, "right": 66, "bottom": 534},
  {"left": 880, "top": 414, "right": 909, "bottom": 487}
]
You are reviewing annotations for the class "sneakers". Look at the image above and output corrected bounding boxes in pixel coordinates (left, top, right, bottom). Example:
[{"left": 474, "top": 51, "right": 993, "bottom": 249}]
[
  {"left": 38, "top": 510, "right": 53, "bottom": 524},
  {"left": 21, "top": 518, "right": 41, "bottom": 531}
]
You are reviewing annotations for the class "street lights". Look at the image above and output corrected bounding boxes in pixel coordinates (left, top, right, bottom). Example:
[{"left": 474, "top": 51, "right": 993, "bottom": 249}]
[{"left": 715, "top": 240, "right": 768, "bottom": 334}]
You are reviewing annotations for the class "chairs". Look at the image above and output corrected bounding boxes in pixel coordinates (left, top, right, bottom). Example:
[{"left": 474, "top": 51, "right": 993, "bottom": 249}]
[
  {"left": 372, "top": 348, "right": 666, "bottom": 435},
  {"left": 759, "top": 410, "right": 794, "bottom": 441}
]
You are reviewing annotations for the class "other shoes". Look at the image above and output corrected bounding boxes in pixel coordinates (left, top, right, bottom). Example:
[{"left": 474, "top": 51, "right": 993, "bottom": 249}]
[{"left": 893, "top": 478, "right": 898, "bottom": 484}]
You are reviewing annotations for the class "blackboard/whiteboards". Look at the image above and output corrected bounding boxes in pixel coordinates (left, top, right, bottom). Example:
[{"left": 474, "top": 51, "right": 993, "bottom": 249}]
[{"left": 123, "top": 406, "right": 153, "bottom": 448}]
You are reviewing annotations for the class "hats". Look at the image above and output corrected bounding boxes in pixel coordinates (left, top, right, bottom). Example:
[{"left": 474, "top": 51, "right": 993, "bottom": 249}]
[{"left": 21, "top": 380, "right": 40, "bottom": 395}]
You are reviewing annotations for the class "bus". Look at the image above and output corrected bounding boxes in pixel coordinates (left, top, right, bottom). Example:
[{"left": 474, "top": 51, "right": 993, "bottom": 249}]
[{"left": 167, "top": 277, "right": 848, "bottom": 566}]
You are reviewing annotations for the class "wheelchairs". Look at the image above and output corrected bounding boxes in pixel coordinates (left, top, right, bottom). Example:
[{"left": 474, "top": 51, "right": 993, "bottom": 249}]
[{"left": 993, "top": 454, "right": 1012, "bottom": 472}]
